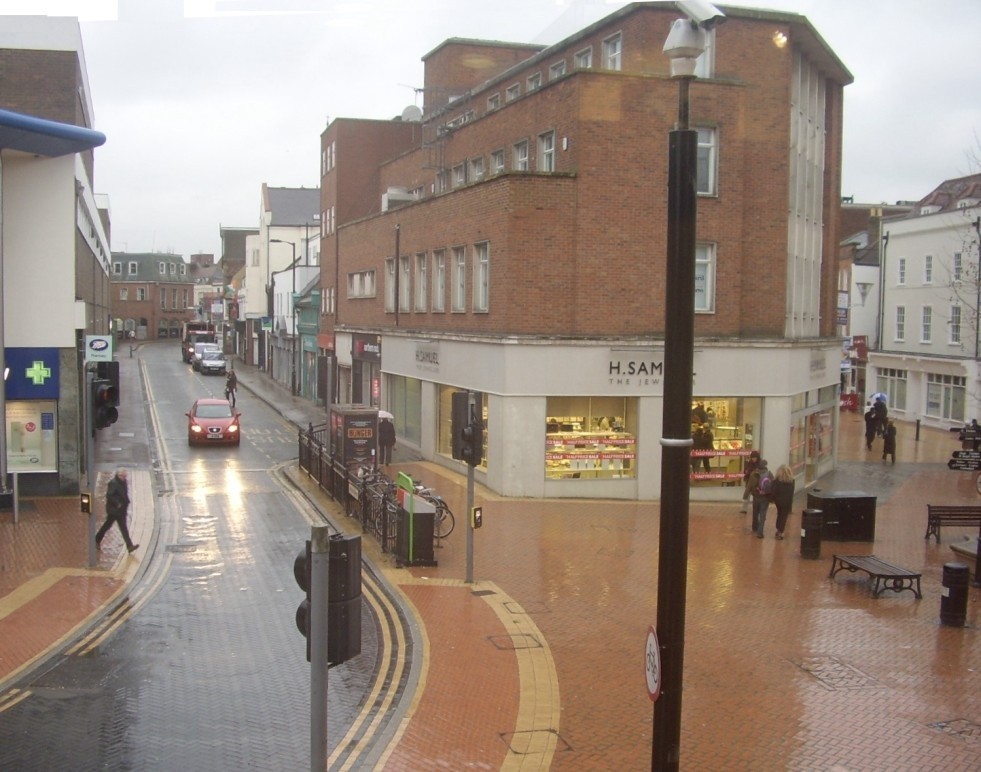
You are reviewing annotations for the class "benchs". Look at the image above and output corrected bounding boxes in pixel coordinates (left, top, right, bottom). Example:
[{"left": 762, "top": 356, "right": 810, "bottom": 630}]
[
  {"left": 924, "top": 504, "right": 981, "bottom": 544},
  {"left": 831, "top": 554, "right": 924, "bottom": 599}
]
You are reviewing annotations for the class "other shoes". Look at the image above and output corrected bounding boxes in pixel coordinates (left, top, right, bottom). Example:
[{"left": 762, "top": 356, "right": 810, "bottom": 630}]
[
  {"left": 129, "top": 544, "right": 139, "bottom": 552},
  {"left": 740, "top": 511, "right": 747, "bottom": 513},
  {"left": 776, "top": 536, "right": 783, "bottom": 540},
  {"left": 757, "top": 531, "right": 762, "bottom": 537},
  {"left": 96, "top": 540, "right": 100, "bottom": 550}
]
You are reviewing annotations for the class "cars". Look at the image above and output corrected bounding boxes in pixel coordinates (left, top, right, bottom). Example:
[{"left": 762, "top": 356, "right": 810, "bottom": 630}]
[{"left": 185, "top": 398, "right": 243, "bottom": 448}]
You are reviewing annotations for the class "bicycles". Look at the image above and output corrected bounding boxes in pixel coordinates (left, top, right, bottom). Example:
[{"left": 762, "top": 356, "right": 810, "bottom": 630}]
[{"left": 351, "top": 455, "right": 456, "bottom": 540}]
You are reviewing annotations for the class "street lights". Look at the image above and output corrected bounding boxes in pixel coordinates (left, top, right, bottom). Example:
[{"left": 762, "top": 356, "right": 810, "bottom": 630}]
[{"left": 270, "top": 239, "right": 297, "bottom": 396}]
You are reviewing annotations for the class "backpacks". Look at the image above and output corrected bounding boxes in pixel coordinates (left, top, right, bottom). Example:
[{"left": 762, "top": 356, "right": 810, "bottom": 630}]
[{"left": 758, "top": 470, "right": 773, "bottom": 494}]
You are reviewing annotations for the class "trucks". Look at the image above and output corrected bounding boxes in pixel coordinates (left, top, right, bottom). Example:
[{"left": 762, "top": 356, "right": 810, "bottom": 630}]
[{"left": 180, "top": 322, "right": 218, "bottom": 363}]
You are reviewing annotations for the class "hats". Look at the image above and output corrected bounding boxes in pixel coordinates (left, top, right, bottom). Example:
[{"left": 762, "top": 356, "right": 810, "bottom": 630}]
[{"left": 751, "top": 450, "right": 759, "bottom": 457}]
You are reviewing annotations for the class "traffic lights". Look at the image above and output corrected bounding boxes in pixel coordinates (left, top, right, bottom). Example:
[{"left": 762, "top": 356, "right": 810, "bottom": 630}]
[
  {"left": 460, "top": 422, "right": 483, "bottom": 466},
  {"left": 91, "top": 377, "right": 118, "bottom": 429},
  {"left": 294, "top": 530, "right": 363, "bottom": 664}
]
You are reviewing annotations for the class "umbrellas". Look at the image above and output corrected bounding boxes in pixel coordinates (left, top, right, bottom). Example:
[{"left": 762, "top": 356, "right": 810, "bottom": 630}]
[
  {"left": 869, "top": 393, "right": 888, "bottom": 399},
  {"left": 377, "top": 410, "right": 394, "bottom": 418}
]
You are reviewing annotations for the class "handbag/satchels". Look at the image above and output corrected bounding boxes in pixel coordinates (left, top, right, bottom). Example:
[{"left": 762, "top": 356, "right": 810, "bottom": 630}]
[{"left": 883, "top": 428, "right": 889, "bottom": 438}]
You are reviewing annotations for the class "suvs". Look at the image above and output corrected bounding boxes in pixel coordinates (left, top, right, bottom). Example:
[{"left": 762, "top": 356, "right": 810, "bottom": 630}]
[{"left": 189, "top": 342, "right": 230, "bottom": 376}]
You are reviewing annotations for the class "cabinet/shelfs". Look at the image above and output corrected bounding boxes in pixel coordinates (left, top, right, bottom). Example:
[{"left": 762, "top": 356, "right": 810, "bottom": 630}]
[
  {"left": 545, "top": 433, "right": 631, "bottom": 480},
  {"left": 709, "top": 426, "right": 745, "bottom": 475}
]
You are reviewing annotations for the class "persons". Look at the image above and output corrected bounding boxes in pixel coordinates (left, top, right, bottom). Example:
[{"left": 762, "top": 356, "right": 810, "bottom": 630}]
[
  {"left": 378, "top": 419, "right": 396, "bottom": 465},
  {"left": 874, "top": 398, "right": 888, "bottom": 438},
  {"left": 95, "top": 468, "right": 139, "bottom": 553},
  {"left": 882, "top": 420, "right": 897, "bottom": 464},
  {"left": 769, "top": 464, "right": 795, "bottom": 539},
  {"left": 691, "top": 401, "right": 715, "bottom": 474},
  {"left": 739, "top": 451, "right": 761, "bottom": 514},
  {"left": 743, "top": 460, "right": 774, "bottom": 538},
  {"left": 864, "top": 402, "right": 878, "bottom": 451},
  {"left": 225, "top": 369, "right": 237, "bottom": 408},
  {"left": 129, "top": 330, "right": 136, "bottom": 343},
  {"left": 959, "top": 419, "right": 981, "bottom": 452}
]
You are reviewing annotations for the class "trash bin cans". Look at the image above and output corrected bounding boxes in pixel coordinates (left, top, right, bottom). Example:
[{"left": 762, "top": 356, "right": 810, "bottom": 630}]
[
  {"left": 397, "top": 489, "right": 436, "bottom": 560},
  {"left": 940, "top": 561, "right": 969, "bottom": 627},
  {"left": 800, "top": 510, "right": 823, "bottom": 561}
]
